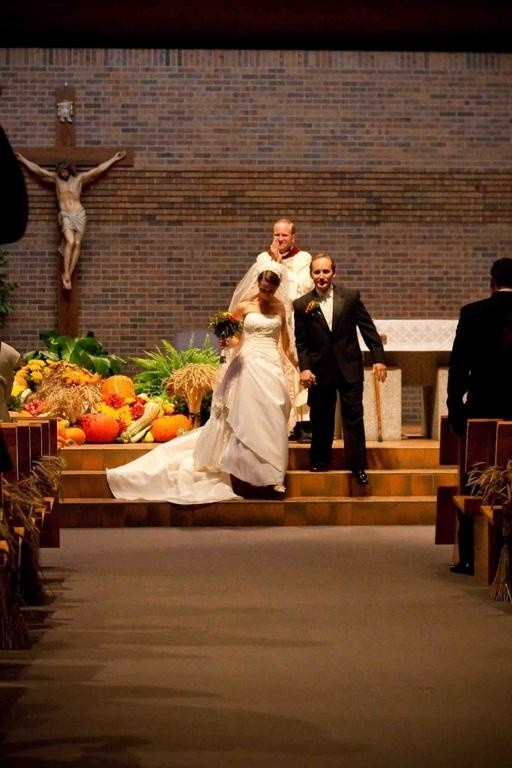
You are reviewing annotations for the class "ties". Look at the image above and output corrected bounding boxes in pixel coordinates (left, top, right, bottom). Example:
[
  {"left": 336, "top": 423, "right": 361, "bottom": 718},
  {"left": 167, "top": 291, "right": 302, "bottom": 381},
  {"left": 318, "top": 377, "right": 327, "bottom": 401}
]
[{"left": 321, "top": 295, "right": 331, "bottom": 331}]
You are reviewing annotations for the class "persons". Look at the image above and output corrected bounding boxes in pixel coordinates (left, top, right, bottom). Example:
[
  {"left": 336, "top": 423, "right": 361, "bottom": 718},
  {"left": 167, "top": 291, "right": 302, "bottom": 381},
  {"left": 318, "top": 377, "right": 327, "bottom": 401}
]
[
  {"left": 253, "top": 214, "right": 317, "bottom": 442},
  {"left": 444, "top": 255, "right": 511, "bottom": 576},
  {"left": 214, "top": 267, "right": 316, "bottom": 498},
  {"left": 291, "top": 251, "right": 390, "bottom": 486},
  {"left": 14, "top": 147, "right": 128, "bottom": 292}
]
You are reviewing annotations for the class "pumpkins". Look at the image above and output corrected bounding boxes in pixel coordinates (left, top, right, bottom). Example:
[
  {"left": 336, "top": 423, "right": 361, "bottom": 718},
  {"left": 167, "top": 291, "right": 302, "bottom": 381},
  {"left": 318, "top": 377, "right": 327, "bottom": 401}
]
[{"left": 57, "top": 375, "right": 192, "bottom": 448}]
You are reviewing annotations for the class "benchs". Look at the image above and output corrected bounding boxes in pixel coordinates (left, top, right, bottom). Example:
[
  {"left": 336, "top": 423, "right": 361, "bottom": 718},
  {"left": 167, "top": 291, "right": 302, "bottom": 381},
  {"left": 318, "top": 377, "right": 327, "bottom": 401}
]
[
  {"left": 434, "top": 416, "right": 512, "bottom": 603},
  {"left": 0, "top": 416, "right": 60, "bottom": 650}
]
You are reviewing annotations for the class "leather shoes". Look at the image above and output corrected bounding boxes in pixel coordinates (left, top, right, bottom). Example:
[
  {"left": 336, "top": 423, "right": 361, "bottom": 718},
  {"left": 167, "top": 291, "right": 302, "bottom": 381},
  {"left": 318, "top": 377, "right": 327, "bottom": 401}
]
[
  {"left": 449, "top": 561, "right": 474, "bottom": 576},
  {"left": 352, "top": 467, "right": 368, "bottom": 485},
  {"left": 312, "top": 465, "right": 328, "bottom": 471},
  {"left": 273, "top": 485, "right": 286, "bottom": 493}
]
